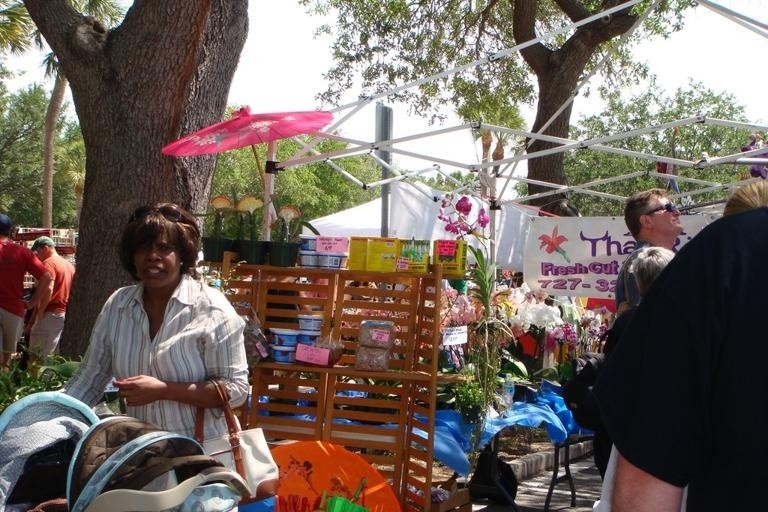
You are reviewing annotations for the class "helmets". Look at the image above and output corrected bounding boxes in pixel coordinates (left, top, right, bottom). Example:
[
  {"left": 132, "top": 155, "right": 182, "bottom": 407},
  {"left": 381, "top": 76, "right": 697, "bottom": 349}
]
[{"left": 31, "top": 236, "right": 54, "bottom": 251}]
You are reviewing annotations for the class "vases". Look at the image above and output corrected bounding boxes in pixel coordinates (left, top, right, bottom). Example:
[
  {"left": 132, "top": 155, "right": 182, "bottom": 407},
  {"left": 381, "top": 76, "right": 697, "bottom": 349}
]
[{"left": 481, "top": 411, "right": 500, "bottom": 454}]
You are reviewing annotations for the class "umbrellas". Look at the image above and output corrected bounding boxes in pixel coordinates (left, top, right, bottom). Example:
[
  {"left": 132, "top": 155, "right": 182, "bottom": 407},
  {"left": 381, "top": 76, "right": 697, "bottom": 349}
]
[{"left": 161, "top": 104, "right": 334, "bottom": 189}]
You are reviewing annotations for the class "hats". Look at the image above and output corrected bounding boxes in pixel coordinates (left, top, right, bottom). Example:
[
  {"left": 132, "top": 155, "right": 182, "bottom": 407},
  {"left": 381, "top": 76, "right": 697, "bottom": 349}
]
[{"left": 0, "top": 214, "right": 13, "bottom": 233}]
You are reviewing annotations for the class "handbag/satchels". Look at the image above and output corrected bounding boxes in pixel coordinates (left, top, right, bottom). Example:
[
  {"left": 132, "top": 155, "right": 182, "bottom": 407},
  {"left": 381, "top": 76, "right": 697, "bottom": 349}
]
[{"left": 190, "top": 376, "right": 281, "bottom": 506}]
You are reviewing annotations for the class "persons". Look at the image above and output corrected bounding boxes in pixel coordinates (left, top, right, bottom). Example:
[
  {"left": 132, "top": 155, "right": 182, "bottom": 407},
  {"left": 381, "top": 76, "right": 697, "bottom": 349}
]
[
  {"left": 591, "top": 245, "right": 678, "bottom": 479},
  {"left": 722, "top": 179, "right": 768, "bottom": 216},
  {"left": 615, "top": 186, "right": 685, "bottom": 318},
  {"left": 0, "top": 212, "right": 51, "bottom": 375},
  {"left": 582, "top": 204, "right": 768, "bottom": 512},
  {"left": 62, "top": 201, "right": 251, "bottom": 444},
  {"left": 27, "top": 236, "right": 75, "bottom": 370}
]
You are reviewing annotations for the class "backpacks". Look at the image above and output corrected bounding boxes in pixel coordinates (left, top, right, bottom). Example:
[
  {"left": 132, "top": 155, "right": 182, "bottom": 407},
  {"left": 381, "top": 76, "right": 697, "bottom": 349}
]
[{"left": 562, "top": 348, "right": 606, "bottom": 433}]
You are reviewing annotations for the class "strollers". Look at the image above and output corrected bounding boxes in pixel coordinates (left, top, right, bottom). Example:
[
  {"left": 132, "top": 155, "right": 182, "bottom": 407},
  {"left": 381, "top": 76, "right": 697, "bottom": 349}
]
[
  {"left": 65, "top": 416, "right": 253, "bottom": 512},
  {"left": 0, "top": 391, "right": 101, "bottom": 512}
]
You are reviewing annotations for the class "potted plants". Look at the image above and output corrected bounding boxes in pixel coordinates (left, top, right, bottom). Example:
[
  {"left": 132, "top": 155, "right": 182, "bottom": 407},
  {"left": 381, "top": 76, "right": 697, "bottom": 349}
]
[{"left": 455, "top": 379, "right": 486, "bottom": 423}]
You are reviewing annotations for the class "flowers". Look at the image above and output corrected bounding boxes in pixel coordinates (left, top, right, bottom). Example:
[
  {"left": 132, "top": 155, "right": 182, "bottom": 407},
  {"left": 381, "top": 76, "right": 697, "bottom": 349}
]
[
  {"left": 342, "top": 276, "right": 611, "bottom": 368},
  {"left": 431, "top": 187, "right": 514, "bottom": 405},
  {"left": 538, "top": 224, "right": 571, "bottom": 264}
]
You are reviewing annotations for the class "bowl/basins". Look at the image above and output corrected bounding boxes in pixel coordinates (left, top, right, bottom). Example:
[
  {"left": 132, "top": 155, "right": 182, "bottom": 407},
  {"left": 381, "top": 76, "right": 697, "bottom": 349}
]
[
  {"left": 298, "top": 236, "right": 349, "bottom": 269},
  {"left": 265, "top": 312, "right": 323, "bottom": 364}
]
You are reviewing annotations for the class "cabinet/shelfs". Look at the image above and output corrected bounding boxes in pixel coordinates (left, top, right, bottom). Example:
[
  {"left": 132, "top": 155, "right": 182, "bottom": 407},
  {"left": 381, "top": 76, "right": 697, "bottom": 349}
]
[{"left": 215, "top": 251, "right": 443, "bottom": 512}]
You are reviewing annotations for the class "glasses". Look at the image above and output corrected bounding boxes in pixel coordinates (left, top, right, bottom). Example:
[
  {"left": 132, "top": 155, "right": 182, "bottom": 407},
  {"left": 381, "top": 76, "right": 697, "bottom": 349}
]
[{"left": 644, "top": 203, "right": 677, "bottom": 215}]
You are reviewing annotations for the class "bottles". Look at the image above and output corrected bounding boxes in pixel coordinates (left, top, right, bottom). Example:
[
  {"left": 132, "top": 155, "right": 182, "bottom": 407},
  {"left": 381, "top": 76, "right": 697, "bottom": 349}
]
[
  {"left": 502, "top": 374, "right": 515, "bottom": 411},
  {"left": 400, "top": 236, "right": 428, "bottom": 263}
]
